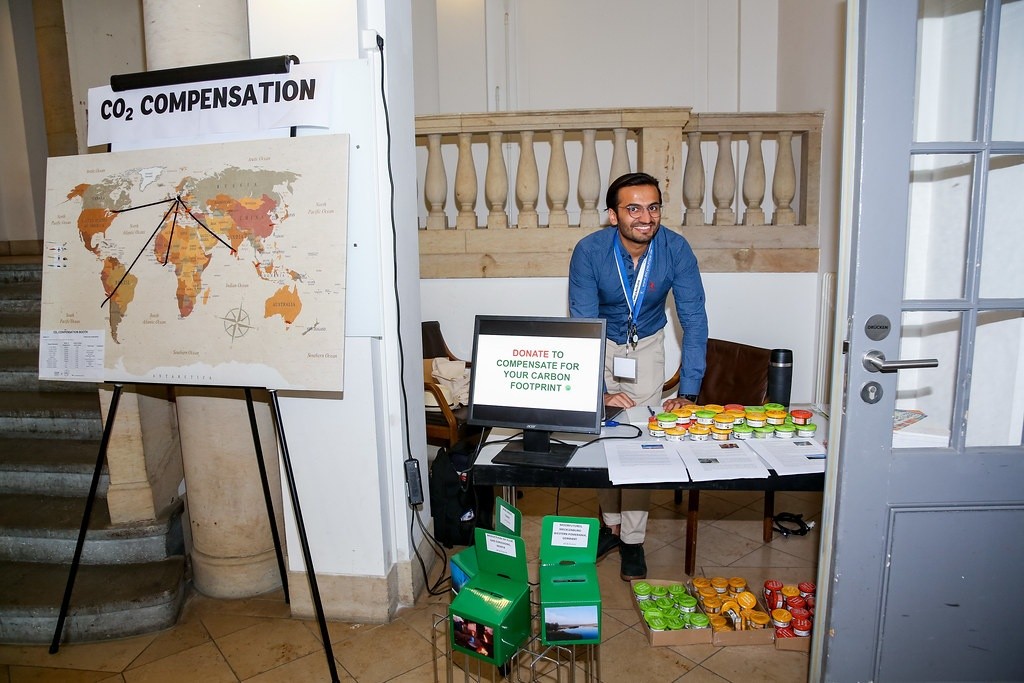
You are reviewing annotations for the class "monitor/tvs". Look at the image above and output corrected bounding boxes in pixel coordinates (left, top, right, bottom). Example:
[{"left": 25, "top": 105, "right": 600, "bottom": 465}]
[{"left": 468, "top": 315, "right": 607, "bottom": 467}]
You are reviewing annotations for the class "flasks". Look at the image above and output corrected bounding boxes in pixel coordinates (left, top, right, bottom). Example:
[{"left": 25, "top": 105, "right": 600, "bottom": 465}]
[{"left": 768, "top": 349, "right": 793, "bottom": 407}]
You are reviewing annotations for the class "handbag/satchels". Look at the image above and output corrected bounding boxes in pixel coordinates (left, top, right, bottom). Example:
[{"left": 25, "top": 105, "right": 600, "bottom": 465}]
[{"left": 428, "top": 433, "right": 494, "bottom": 549}]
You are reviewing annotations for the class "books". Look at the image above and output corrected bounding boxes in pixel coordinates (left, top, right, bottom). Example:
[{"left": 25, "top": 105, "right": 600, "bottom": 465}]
[{"left": 601, "top": 406, "right": 624, "bottom": 427}]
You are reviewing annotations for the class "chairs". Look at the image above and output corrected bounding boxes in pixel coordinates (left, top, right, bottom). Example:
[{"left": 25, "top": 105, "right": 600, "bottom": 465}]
[
  {"left": 697, "top": 339, "right": 775, "bottom": 404},
  {"left": 420, "top": 321, "right": 489, "bottom": 453}
]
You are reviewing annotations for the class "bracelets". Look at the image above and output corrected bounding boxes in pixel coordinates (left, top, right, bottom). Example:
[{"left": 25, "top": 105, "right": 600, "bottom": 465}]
[{"left": 679, "top": 394, "right": 697, "bottom": 402}]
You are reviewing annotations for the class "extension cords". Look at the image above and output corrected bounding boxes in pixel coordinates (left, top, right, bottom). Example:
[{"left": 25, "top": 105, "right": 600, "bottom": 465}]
[{"left": 406, "top": 458, "right": 424, "bottom": 506}]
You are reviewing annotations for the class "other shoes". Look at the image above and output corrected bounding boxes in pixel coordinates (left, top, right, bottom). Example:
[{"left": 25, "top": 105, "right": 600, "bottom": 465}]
[
  {"left": 595, "top": 527, "right": 619, "bottom": 562},
  {"left": 619, "top": 538, "right": 647, "bottom": 581}
]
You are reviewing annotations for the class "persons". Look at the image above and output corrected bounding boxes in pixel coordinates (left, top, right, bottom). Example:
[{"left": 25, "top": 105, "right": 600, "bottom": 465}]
[{"left": 568, "top": 172, "right": 708, "bottom": 581}]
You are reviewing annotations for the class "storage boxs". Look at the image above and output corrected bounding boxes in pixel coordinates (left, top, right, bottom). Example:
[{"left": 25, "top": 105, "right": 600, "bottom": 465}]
[
  {"left": 629, "top": 577, "right": 712, "bottom": 646},
  {"left": 447, "top": 495, "right": 602, "bottom": 668},
  {"left": 761, "top": 583, "right": 812, "bottom": 653},
  {"left": 689, "top": 579, "right": 774, "bottom": 646}
]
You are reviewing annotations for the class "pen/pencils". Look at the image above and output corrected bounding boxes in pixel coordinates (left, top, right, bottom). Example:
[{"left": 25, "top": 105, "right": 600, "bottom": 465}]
[{"left": 647, "top": 405, "right": 655, "bottom": 416}]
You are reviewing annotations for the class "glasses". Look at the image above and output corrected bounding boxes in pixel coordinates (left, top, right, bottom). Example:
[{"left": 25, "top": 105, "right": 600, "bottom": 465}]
[{"left": 617, "top": 204, "right": 664, "bottom": 218}]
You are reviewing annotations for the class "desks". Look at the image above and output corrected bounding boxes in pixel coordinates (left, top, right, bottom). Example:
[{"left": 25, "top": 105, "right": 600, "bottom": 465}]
[{"left": 474, "top": 403, "right": 831, "bottom": 574}]
